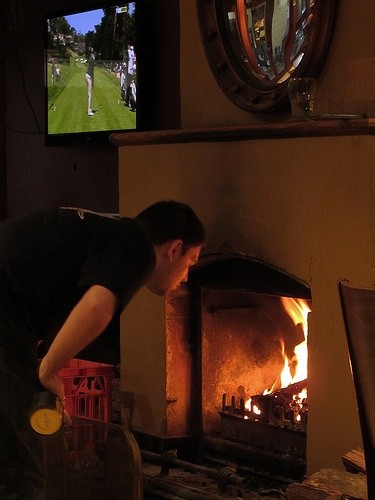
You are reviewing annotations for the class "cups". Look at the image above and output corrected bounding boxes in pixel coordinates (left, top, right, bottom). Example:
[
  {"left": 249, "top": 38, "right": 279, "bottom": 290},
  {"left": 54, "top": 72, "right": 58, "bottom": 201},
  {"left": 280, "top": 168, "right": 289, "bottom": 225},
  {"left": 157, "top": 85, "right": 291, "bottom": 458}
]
[{"left": 288, "top": 78, "right": 315, "bottom": 120}]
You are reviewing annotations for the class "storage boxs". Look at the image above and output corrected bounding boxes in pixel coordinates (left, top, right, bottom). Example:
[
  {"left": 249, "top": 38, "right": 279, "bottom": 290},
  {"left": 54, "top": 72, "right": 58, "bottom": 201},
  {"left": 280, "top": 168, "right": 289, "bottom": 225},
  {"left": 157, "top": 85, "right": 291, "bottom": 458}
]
[{"left": 38, "top": 358, "right": 116, "bottom": 460}]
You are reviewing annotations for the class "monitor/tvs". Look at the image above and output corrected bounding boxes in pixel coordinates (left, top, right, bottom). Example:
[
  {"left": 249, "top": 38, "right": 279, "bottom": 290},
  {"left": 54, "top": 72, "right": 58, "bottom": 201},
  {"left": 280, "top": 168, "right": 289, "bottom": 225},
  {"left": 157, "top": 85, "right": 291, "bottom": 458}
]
[{"left": 42, "top": 0, "right": 142, "bottom": 138}]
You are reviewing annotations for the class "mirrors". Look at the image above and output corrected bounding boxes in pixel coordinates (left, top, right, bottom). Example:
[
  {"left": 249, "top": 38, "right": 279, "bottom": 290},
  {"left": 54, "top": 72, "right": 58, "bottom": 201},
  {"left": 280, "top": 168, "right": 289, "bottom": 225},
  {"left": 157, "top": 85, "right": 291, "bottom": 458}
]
[{"left": 196, "top": 0, "right": 340, "bottom": 113}]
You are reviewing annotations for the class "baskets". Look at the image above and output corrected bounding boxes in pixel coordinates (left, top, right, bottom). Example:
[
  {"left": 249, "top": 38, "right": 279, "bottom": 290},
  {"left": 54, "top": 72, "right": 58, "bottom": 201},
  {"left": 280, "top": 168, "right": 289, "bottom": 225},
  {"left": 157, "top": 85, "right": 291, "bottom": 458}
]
[{"left": 55, "top": 358, "right": 116, "bottom": 424}]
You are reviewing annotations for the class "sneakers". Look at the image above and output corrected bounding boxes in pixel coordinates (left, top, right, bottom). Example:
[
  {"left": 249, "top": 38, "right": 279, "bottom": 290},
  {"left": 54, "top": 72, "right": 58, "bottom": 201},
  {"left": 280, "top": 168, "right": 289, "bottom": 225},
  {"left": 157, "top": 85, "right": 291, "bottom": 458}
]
[{"left": 88, "top": 111, "right": 95, "bottom": 116}]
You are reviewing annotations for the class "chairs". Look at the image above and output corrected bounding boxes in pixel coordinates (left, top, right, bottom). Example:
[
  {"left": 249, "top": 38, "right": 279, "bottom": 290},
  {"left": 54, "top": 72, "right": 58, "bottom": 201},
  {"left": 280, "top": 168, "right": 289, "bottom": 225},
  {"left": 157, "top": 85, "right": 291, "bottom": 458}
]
[{"left": 336, "top": 277, "right": 375, "bottom": 500}]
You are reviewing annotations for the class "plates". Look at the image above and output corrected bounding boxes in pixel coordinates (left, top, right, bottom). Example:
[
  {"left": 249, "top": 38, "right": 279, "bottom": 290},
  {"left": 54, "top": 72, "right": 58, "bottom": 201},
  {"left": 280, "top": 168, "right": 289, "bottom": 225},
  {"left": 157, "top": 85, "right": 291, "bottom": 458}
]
[{"left": 309, "top": 114, "right": 360, "bottom": 120}]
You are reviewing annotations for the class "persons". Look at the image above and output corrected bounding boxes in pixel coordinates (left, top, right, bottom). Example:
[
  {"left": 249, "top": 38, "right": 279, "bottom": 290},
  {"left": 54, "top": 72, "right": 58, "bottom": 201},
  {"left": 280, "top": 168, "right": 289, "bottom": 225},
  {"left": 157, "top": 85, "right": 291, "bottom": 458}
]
[
  {"left": 51, "top": 44, "right": 136, "bottom": 116},
  {"left": 0, "top": 201, "right": 206, "bottom": 500}
]
